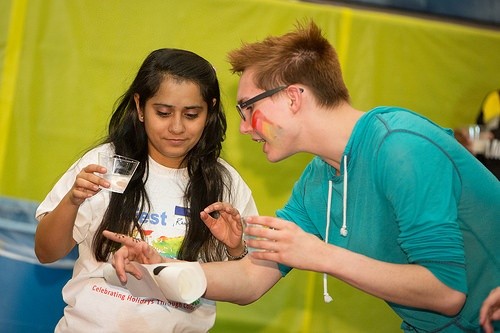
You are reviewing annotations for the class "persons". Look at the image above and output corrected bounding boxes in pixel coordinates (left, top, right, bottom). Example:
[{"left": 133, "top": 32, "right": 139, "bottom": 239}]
[
  {"left": 479, "top": 284, "right": 500, "bottom": 333},
  {"left": 103, "top": 15, "right": 500, "bottom": 333},
  {"left": 33, "top": 48, "right": 265, "bottom": 333}
]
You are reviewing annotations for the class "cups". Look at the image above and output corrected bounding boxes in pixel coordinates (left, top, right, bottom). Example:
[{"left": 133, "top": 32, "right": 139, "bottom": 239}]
[
  {"left": 241, "top": 215, "right": 275, "bottom": 254},
  {"left": 96, "top": 152, "right": 141, "bottom": 194}
]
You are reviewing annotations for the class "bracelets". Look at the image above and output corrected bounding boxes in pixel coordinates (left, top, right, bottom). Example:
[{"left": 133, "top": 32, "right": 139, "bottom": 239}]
[{"left": 225, "top": 242, "right": 248, "bottom": 260}]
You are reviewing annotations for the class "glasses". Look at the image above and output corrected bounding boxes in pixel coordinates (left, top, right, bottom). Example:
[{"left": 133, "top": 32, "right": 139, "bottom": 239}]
[{"left": 236, "top": 85, "right": 304, "bottom": 121}]
